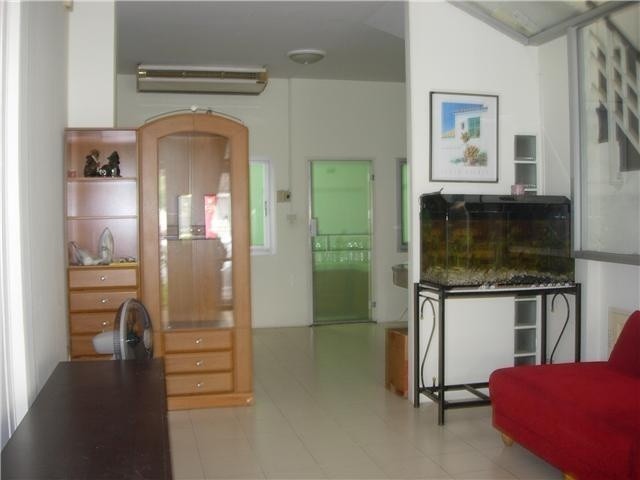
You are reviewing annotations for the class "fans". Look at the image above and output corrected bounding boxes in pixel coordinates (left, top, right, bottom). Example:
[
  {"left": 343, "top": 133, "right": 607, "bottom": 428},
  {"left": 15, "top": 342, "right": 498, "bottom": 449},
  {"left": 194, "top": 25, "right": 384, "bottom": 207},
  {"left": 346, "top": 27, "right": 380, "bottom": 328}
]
[{"left": 92, "top": 297, "right": 153, "bottom": 361}]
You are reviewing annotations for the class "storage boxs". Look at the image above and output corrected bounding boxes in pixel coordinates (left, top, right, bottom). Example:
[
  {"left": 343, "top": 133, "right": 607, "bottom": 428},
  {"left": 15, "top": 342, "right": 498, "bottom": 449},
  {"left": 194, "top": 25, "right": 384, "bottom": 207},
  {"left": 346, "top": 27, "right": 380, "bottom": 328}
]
[{"left": 384, "top": 327, "right": 408, "bottom": 400}]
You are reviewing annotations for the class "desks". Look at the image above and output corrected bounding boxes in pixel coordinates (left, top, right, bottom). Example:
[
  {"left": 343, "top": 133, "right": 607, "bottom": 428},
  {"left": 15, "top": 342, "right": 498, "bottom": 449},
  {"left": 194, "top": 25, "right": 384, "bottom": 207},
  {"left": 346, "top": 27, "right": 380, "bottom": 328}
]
[
  {"left": 0, "top": 358, "right": 173, "bottom": 480},
  {"left": 414, "top": 283, "right": 582, "bottom": 426}
]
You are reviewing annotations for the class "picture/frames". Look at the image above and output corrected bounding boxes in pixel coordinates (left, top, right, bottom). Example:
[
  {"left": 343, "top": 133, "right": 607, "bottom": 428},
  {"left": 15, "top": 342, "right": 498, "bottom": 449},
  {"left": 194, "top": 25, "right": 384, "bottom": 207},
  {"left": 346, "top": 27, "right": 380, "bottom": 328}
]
[{"left": 429, "top": 92, "right": 499, "bottom": 183}]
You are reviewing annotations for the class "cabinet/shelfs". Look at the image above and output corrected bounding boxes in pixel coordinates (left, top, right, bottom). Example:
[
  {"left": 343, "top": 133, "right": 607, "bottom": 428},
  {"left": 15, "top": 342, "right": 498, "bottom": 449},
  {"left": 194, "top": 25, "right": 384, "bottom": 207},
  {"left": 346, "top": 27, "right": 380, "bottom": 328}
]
[
  {"left": 63, "top": 128, "right": 141, "bottom": 361},
  {"left": 514, "top": 160, "right": 537, "bottom": 192},
  {"left": 139, "top": 107, "right": 255, "bottom": 411}
]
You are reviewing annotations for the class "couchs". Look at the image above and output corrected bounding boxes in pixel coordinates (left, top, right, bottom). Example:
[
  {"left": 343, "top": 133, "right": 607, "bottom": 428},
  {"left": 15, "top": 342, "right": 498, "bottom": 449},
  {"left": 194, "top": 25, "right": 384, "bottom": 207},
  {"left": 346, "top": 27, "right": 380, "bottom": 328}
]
[{"left": 489, "top": 310, "right": 640, "bottom": 480}]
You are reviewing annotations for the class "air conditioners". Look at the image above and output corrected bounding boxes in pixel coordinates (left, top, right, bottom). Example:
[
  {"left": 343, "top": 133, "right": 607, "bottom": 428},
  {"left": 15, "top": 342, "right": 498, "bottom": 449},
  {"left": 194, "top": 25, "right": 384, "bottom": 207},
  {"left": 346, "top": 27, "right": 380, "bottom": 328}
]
[{"left": 137, "top": 63, "right": 268, "bottom": 94}]
